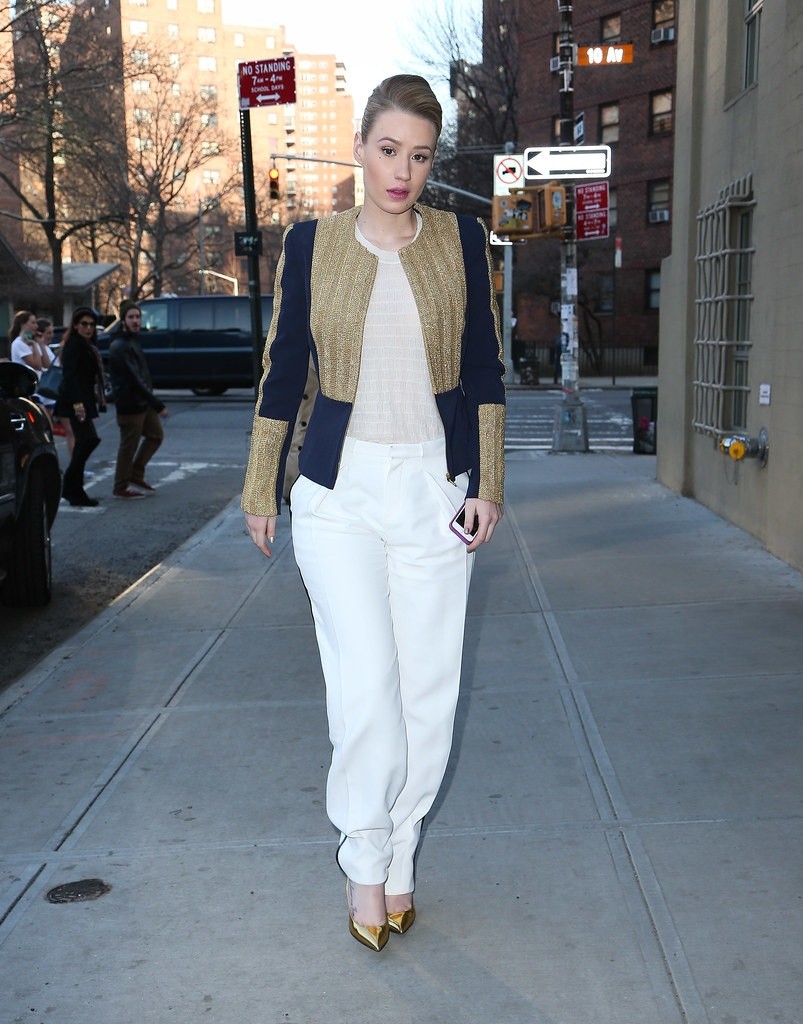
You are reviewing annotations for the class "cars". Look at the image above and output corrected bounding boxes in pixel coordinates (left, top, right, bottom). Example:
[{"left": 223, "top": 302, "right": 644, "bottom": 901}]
[
  {"left": 1, "top": 357, "right": 63, "bottom": 607},
  {"left": 48, "top": 324, "right": 105, "bottom": 356}
]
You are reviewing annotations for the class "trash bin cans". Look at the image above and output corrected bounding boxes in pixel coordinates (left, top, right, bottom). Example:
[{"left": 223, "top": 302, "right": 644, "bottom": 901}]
[{"left": 629, "top": 384, "right": 660, "bottom": 454}]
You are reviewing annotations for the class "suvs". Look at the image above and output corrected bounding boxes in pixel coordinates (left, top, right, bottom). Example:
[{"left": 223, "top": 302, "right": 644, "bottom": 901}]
[{"left": 94, "top": 292, "right": 273, "bottom": 404}]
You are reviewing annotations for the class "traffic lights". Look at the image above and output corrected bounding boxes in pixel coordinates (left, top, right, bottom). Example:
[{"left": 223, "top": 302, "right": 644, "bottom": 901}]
[
  {"left": 536, "top": 186, "right": 567, "bottom": 232},
  {"left": 233, "top": 230, "right": 264, "bottom": 257},
  {"left": 269, "top": 168, "right": 280, "bottom": 201}
]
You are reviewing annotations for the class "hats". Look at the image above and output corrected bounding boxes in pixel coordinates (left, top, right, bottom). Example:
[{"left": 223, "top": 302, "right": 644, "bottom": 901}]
[{"left": 120, "top": 300, "right": 141, "bottom": 321}]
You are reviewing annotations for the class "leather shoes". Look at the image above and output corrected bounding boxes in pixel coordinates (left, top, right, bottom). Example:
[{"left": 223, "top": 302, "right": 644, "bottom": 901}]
[
  {"left": 346, "top": 876, "right": 389, "bottom": 952},
  {"left": 387, "top": 891, "right": 415, "bottom": 935}
]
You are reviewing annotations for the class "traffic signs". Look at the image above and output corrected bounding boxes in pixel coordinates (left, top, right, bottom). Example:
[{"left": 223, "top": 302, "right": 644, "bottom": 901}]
[
  {"left": 573, "top": 112, "right": 586, "bottom": 146},
  {"left": 573, "top": 180, "right": 610, "bottom": 243},
  {"left": 236, "top": 55, "right": 296, "bottom": 111}
]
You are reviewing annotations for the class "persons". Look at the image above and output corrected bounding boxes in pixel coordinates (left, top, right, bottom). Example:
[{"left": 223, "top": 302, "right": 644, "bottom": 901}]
[
  {"left": 9, "top": 310, "right": 95, "bottom": 478},
  {"left": 240, "top": 74, "right": 507, "bottom": 951},
  {"left": 106, "top": 300, "right": 168, "bottom": 499},
  {"left": 51, "top": 307, "right": 107, "bottom": 508}
]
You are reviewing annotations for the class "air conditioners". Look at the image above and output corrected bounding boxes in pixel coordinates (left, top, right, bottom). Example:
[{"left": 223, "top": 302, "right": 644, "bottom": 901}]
[
  {"left": 551, "top": 302, "right": 561, "bottom": 313},
  {"left": 648, "top": 209, "right": 669, "bottom": 223},
  {"left": 550, "top": 55, "right": 560, "bottom": 72},
  {"left": 651, "top": 28, "right": 674, "bottom": 43}
]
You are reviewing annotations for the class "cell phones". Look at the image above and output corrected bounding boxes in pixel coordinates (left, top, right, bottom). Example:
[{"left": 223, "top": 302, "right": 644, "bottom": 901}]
[{"left": 449, "top": 503, "right": 479, "bottom": 545}]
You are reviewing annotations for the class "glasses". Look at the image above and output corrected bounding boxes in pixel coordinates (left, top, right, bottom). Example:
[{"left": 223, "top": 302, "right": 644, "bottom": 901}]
[{"left": 80, "top": 321, "right": 96, "bottom": 328}]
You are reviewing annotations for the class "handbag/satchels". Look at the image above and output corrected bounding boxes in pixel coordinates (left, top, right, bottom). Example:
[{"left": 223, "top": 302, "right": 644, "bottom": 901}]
[{"left": 37, "top": 353, "right": 63, "bottom": 400}]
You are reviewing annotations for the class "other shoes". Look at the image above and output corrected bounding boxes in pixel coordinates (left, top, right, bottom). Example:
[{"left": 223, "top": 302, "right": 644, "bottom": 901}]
[
  {"left": 70, "top": 496, "right": 99, "bottom": 507},
  {"left": 113, "top": 488, "right": 146, "bottom": 499},
  {"left": 132, "top": 479, "right": 156, "bottom": 492},
  {"left": 62, "top": 488, "right": 86, "bottom": 502}
]
